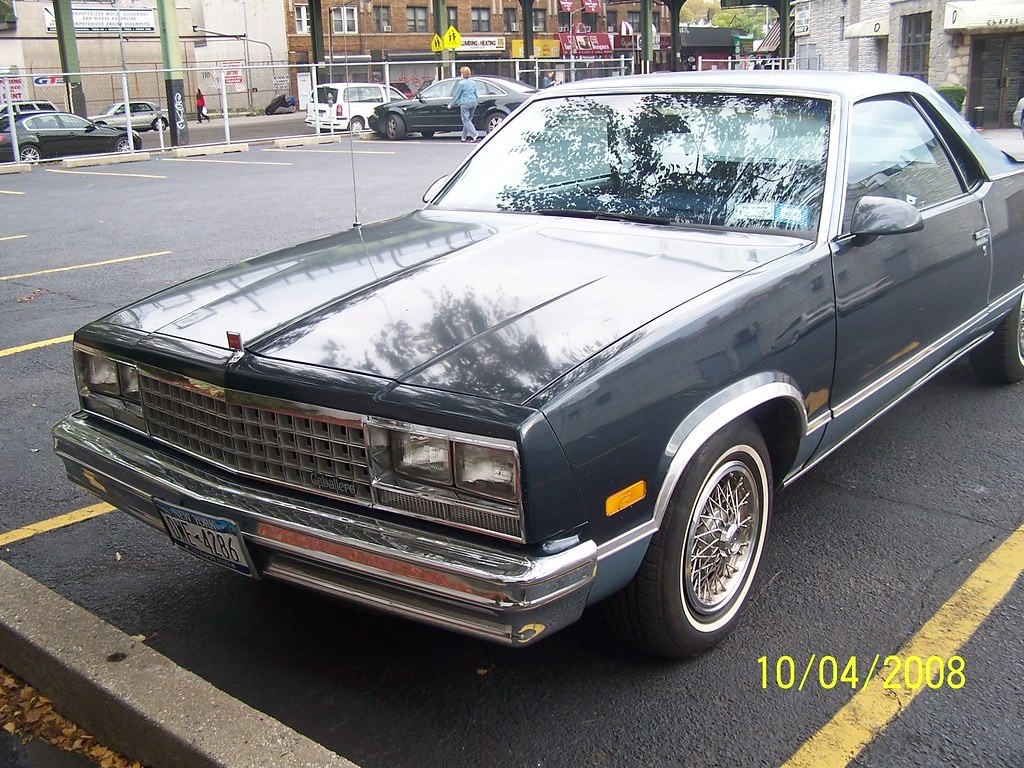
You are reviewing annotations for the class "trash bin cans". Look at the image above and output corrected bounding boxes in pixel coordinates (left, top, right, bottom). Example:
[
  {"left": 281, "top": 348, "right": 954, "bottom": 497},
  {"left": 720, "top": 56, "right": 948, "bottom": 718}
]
[{"left": 973, "top": 106, "right": 985, "bottom": 129}]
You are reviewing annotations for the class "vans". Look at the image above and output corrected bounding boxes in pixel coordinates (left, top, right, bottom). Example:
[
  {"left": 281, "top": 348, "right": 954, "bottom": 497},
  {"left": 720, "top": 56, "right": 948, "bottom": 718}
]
[{"left": 304, "top": 83, "right": 408, "bottom": 133}]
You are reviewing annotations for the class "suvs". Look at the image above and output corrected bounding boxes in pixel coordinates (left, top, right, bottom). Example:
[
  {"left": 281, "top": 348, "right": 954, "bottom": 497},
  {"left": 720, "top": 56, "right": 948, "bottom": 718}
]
[{"left": 1, "top": 100, "right": 59, "bottom": 116}]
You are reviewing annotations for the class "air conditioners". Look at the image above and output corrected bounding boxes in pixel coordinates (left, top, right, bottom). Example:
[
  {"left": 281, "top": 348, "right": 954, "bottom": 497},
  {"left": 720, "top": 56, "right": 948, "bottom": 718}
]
[
  {"left": 607, "top": 26, "right": 613, "bottom": 32},
  {"left": 559, "top": 27, "right": 566, "bottom": 32},
  {"left": 511, "top": 22, "right": 520, "bottom": 32},
  {"left": 383, "top": 26, "right": 392, "bottom": 32},
  {"left": 585, "top": 25, "right": 592, "bottom": 32},
  {"left": 532, "top": 26, "right": 539, "bottom": 32}
]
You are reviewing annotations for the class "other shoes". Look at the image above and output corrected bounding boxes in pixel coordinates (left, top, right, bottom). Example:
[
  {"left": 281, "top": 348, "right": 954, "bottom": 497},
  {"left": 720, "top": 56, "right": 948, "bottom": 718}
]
[
  {"left": 470, "top": 133, "right": 480, "bottom": 143},
  {"left": 460, "top": 139, "right": 466, "bottom": 142}
]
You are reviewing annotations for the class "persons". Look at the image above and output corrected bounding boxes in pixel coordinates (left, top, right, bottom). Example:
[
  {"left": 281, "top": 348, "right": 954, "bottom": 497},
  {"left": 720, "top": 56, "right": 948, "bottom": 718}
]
[
  {"left": 195, "top": 87, "right": 212, "bottom": 125},
  {"left": 541, "top": 71, "right": 559, "bottom": 91},
  {"left": 447, "top": 66, "right": 480, "bottom": 143}
]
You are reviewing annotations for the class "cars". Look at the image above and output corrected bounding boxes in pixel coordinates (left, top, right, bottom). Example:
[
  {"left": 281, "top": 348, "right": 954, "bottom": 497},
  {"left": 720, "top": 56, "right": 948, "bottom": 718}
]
[
  {"left": 0, "top": 112, "right": 143, "bottom": 162},
  {"left": 381, "top": 83, "right": 413, "bottom": 99},
  {"left": 51, "top": 69, "right": 1023, "bottom": 660},
  {"left": 88, "top": 102, "right": 170, "bottom": 131},
  {"left": 368, "top": 75, "right": 540, "bottom": 140}
]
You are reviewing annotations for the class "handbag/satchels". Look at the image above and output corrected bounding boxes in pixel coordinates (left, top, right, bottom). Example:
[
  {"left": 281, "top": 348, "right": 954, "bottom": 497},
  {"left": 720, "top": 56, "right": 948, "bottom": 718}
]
[{"left": 202, "top": 106, "right": 208, "bottom": 116}]
